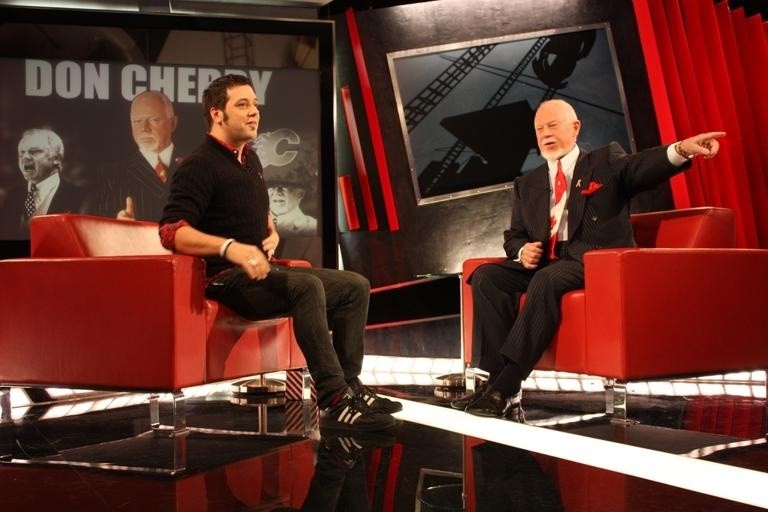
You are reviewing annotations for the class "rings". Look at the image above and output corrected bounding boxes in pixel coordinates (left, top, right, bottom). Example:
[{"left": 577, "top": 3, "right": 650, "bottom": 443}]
[{"left": 247, "top": 257, "right": 257, "bottom": 268}]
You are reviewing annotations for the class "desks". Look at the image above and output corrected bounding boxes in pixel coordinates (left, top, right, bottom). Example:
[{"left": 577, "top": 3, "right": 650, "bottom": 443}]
[
  {"left": 415, "top": 392, "right": 466, "bottom": 511},
  {"left": 416, "top": 273, "right": 462, "bottom": 389}
]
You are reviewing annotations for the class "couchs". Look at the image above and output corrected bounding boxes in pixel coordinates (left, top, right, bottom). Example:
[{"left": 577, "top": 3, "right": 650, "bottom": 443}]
[
  {"left": 1, "top": 209, "right": 316, "bottom": 437},
  {"left": 457, "top": 202, "right": 767, "bottom": 424}
]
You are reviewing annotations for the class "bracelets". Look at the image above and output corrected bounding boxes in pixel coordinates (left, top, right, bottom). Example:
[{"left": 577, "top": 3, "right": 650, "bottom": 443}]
[
  {"left": 678, "top": 140, "right": 691, "bottom": 158},
  {"left": 219, "top": 238, "right": 235, "bottom": 258}
]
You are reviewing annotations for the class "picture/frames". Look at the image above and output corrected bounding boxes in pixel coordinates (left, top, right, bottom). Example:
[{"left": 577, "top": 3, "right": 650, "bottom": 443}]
[{"left": 381, "top": 21, "right": 642, "bottom": 210}]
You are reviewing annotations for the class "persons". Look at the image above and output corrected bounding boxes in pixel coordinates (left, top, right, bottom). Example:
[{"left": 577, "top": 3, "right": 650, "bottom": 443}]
[
  {"left": 449, "top": 96, "right": 728, "bottom": 418},
  {"left": 89, "top": 90, "right": 203, "bottom": 223},
  {"left": 1, "top": 126, "right": 86, "bottom": 241},
  {"left": 157, "top": 72, "right": 404, "bottom": 434},
  {"left": 290, "top": 438, "right": 372, "bottom": 512},
  {"left": 266, "top": 167, "right": 317, "bottom": 239}
]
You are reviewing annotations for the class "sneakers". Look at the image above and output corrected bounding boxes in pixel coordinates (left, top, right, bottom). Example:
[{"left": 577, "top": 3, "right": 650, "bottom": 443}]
[
  {"left": 318, "top": 400, "right": 396, "bottom": 432},
  {"left": 354, "top": 386, "right": 403, "bottom": 415}
]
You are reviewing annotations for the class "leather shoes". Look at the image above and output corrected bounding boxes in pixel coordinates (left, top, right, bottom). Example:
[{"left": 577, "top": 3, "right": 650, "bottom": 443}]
[{"left": 452, "top": 383, "right": 523, "bottom": 416}]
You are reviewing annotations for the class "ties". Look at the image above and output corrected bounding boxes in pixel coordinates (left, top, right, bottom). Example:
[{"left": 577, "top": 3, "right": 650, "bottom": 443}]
[
  {"left": 547, "top": 159, "right": 569, "bottom": 260},
  {"left": 23, "top": 183, "right": 39, "bottom": 217},
  {"left": 155, "top": 160, "right": 167, "bottom": 184}
]
